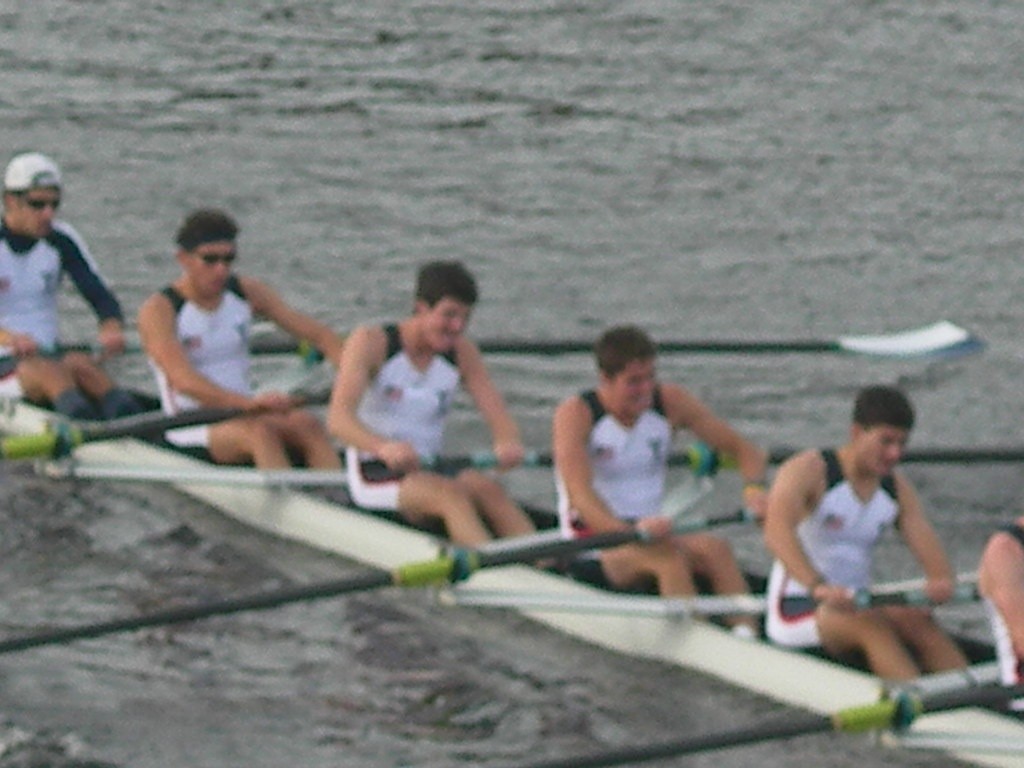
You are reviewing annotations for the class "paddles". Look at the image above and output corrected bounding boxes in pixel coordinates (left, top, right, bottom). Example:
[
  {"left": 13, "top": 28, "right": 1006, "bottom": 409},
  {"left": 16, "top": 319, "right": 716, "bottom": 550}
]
[
  {"left": 0, "top": 377, "right": 332, "bottom": 459},
  {"left": 523, "top": 683, "right": 1024, "bottom": 768},
  {"left": 0, "top": 509, "right": 766, "bottom": 650},
  {"left": 49, "top": 316, "right": 969, "bottom": 365},
  {"left": 24, "top": 449, "right": 351, "bottom": 490},
  {"left": 364, "top": 437, "right": 1024, "bottom": 474}
]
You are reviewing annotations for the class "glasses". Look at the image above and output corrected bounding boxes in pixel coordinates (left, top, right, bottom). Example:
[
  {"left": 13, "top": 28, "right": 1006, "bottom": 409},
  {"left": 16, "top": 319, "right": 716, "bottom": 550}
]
[
  {"left": 19, "top": 193, "right": 60, "bottom": 210},
  {"left": 191, "top": 249, "right": 237, "bottom": 266}
]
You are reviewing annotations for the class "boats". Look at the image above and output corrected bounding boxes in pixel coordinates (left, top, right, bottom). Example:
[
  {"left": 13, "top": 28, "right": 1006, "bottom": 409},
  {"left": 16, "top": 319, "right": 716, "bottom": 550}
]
[{"left": 0, "top": 386, "right": 1024, "bottom": 768}]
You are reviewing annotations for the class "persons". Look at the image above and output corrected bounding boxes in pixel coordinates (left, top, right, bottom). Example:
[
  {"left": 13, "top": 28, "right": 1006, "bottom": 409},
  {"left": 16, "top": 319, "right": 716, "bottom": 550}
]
[
  {"left": 764, "top": 384, "right": 966, "bottom": 680},
  {"left": 137, "top": 210, "right": 344, "bottom": 468},
  {"left": 975, "top": 511, "right": 1024, "bottom": 715},
  {"left": 0, "top": 153, "right": 146, "bottom": 420},
  {"left": 553, "top": 325, "right": 768, "bottom": 638},
  {"left": 326, "top": 263, "right": 552, "bottom": 569}
]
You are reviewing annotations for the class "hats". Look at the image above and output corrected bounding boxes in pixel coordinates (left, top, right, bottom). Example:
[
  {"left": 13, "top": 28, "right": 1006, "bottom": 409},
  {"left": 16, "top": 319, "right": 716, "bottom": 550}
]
[{"left": 3, "top": 153, "right": 63, "bottom": 194}]
